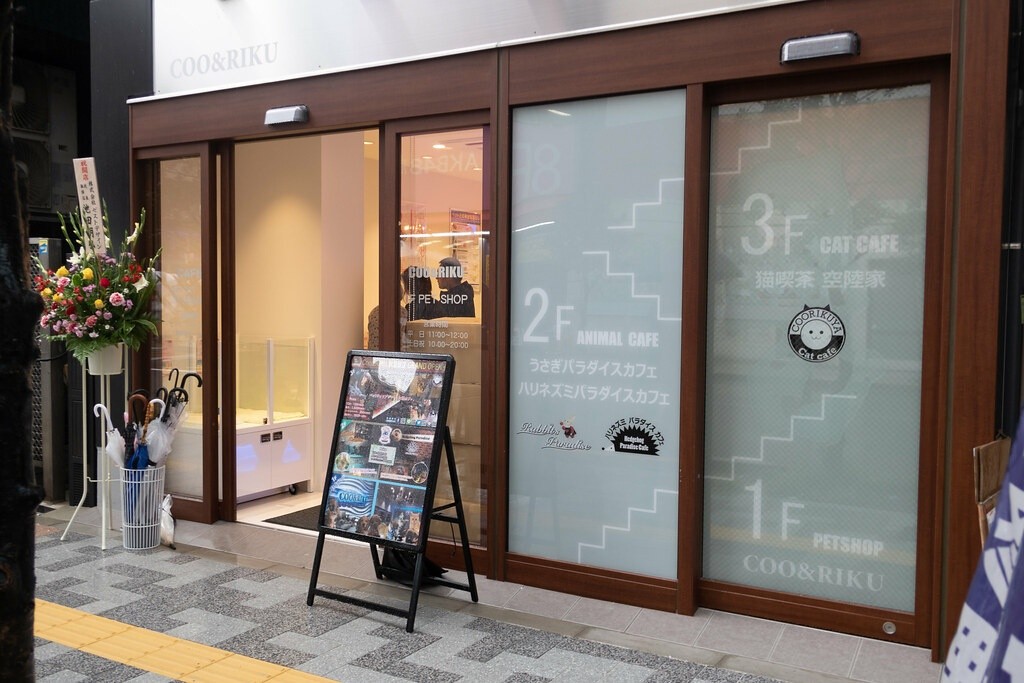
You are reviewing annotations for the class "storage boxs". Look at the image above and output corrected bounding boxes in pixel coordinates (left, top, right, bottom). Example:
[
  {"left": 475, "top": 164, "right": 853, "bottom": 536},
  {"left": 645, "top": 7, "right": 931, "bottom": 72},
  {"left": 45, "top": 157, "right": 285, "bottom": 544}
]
[{"left": 404, "top": 317, "right": 481, "bottom": 541}]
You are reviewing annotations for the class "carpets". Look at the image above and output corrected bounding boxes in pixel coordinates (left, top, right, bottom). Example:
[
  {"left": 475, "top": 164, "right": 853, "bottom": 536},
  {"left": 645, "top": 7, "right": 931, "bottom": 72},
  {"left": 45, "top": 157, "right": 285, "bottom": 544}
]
[{"left": 263, "top": 505, "right": 321, "bottom": 530}]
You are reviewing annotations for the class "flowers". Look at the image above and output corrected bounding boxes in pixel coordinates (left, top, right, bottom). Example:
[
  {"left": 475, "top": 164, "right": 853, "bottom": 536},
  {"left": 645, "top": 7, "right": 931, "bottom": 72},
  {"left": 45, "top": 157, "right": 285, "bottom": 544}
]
[{"left": 30, "top": 197, "right": 165, "bottom": 364}]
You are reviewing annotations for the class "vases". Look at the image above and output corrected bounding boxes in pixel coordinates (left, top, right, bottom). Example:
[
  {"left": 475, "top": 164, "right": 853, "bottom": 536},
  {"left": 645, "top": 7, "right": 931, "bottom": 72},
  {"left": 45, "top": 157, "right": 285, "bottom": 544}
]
[{"left": 88, "top": 342, "right": 123, "bottom": 374}]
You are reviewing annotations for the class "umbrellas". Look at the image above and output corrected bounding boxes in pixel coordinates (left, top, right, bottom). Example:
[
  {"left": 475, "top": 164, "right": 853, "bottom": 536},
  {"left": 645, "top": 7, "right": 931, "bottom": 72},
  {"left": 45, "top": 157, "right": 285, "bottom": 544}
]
[{"left": 93, "top": 366, "right": 204, "bottom": 549}]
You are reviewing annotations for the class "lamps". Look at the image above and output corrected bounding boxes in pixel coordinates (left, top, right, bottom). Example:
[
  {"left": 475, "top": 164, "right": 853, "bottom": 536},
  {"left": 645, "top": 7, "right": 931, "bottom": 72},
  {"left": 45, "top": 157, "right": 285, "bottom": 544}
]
[
  {"left": 263, "top": 105, "right": 309, "bottom": 125},
  {"left": 780, "top": 32, "right": 858, "bottom": 63}
]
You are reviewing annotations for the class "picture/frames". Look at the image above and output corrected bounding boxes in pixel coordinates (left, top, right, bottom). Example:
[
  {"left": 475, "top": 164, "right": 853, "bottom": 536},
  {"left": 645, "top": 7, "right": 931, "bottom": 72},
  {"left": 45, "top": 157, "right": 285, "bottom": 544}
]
[{"left": 448, "top": 207, "right": 481, "bottom": 293}]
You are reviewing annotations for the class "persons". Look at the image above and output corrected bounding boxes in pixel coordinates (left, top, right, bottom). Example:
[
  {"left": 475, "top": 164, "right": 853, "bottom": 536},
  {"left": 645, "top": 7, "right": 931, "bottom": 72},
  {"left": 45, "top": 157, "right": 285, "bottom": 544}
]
[
  {"left": 367, "top": 282, "right": 408, "bottom": 352},
  {"left": 400, "top": 264, "right": 449, "bottom": 322},
  {"left": 435, "top": 257, "right": 476, "bottom": 318}
]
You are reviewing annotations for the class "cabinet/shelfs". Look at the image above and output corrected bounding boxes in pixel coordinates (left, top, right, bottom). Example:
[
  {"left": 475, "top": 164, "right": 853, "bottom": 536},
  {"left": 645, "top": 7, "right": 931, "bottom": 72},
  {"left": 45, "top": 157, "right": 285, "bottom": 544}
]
[{"left": 161, "top": 336, "right": 314, "bottom": 505}]
